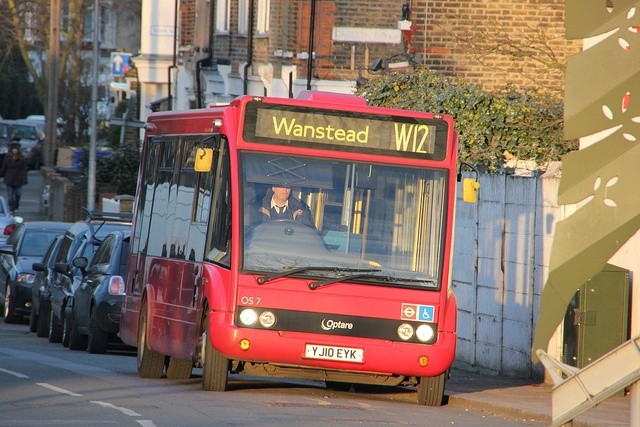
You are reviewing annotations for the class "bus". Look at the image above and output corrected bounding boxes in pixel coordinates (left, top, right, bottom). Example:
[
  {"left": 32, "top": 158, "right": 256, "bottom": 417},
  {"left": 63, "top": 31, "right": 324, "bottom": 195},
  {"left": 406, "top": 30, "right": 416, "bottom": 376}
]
[{"left": 121, "top": 95, "right": 480, "bottom": 405}]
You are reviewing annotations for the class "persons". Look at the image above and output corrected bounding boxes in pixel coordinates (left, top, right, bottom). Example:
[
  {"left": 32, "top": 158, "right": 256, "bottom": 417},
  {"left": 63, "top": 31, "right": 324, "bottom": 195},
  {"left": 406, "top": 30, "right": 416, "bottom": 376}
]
[
  {"left": 258, "top": 184, "right": 317, "bottom": 227},
  {"left": 1, "top": 143, "right": 28, "bottom": 216}
]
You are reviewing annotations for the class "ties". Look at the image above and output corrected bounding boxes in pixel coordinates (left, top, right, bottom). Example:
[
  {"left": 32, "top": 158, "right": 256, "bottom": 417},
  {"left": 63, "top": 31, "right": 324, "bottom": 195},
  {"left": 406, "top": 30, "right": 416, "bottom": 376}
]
[{"left": 276, "top": 205, "right": 286, "bottom": 214}]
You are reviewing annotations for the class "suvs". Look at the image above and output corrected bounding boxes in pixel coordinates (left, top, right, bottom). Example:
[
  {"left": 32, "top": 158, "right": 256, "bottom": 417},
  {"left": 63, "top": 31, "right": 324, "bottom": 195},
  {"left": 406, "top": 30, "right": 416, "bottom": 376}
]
[{"left": 54, "top": 220, "right": 131, "bottom": 344}]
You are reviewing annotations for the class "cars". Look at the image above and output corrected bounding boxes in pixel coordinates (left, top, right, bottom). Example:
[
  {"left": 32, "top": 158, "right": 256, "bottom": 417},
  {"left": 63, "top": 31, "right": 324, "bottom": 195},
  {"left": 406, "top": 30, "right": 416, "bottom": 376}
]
[
  {"left": 29, "top": 234, "right": 63, "bottom": 338},
  {"left": 0, "top": 195, "right": 23, "bottom": 245},
  {"left": 11, "top": 121, "right": 45, "bottom": 170},
  {"left": 75, "top": 230, "right": 136, "bottom": 349},
  {"left": 3, "top": 221, "right": 73, "bottom": 323},
  {"left": 0, "top": 121, "right": 14, "bottom": 158}
]
